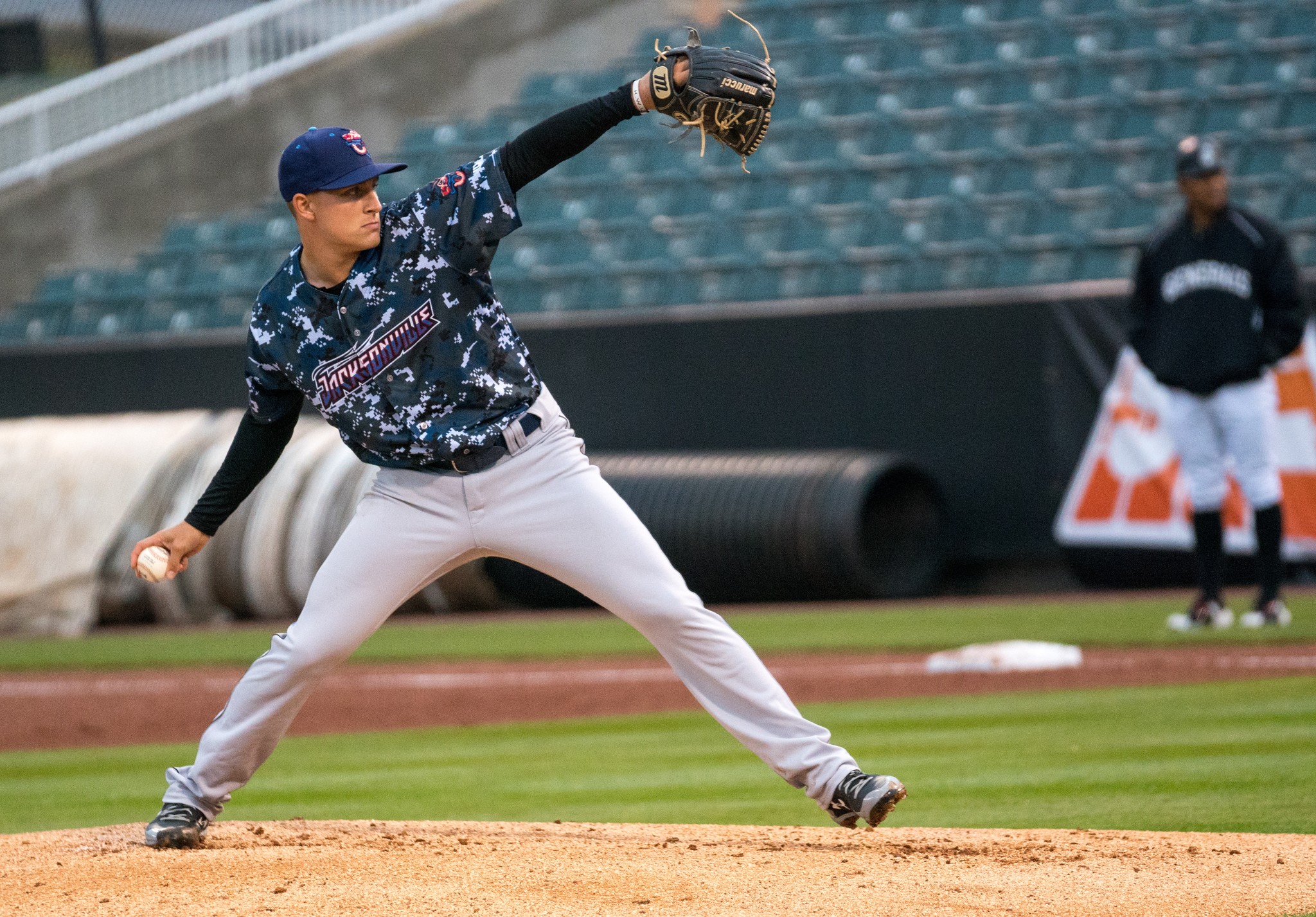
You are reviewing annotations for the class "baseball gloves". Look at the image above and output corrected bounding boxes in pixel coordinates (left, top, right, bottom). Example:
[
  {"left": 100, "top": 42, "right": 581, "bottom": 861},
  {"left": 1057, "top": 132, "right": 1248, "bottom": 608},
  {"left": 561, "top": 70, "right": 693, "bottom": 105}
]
[{"left": 649, "top": 42, "right": 778, "bottom": 159}]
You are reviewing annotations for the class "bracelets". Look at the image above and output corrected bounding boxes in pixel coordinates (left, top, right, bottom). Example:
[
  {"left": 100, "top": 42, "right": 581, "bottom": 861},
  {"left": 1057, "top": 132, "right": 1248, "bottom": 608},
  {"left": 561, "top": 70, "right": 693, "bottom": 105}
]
[{"left": 633, "top": 79, "right": 650, "bottom": 115}]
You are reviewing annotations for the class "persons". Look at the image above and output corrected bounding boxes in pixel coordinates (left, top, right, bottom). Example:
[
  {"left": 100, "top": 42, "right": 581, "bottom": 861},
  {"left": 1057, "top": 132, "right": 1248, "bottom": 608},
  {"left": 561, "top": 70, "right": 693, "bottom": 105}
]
[
  {"left": 131, "top": 10, "right": 906, "bottom": 847},
  {"left": 1124, "top": 133, "right": 1309, "bottom": 633}
]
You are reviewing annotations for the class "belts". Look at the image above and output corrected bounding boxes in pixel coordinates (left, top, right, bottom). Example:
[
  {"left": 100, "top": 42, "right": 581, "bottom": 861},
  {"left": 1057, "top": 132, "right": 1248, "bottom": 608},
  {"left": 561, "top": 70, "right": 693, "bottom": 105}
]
[{"left": 425, "top": 413, "right": 541, "bottom": 477}]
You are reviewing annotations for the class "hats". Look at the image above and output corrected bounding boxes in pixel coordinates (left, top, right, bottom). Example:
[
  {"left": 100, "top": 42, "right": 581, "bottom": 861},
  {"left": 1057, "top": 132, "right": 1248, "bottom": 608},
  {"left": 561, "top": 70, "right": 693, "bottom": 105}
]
[
  {"left": 279, "top": 126, "right": 407, "bottom": 201},
  {"left": 1176, "top": 137, "right": 1222, "bottom": 179}
]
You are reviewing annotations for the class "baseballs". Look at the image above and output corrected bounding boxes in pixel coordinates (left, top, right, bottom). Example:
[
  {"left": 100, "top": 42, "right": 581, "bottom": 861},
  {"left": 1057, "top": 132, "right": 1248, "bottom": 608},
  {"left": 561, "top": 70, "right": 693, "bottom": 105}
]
[{"left": 136, "top": 546, "right": 173, "bottom": 583}]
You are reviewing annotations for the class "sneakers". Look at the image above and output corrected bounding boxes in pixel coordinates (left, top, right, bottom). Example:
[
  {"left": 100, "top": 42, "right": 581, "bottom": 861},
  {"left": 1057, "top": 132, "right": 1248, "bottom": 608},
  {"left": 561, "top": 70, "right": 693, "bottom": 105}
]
[
  {"left": 144, "top": 802, "right": 208, "bottom": 849},
  {"left": 828, "top": 769, "right": 907, "bottom": 829}
]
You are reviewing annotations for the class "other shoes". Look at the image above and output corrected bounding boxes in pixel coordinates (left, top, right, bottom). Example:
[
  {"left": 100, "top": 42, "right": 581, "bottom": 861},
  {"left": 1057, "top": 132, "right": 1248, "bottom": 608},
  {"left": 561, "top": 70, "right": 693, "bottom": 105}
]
[
  {"left": 1239, "top": 599, "right": 1288, "bottom": 625},
  {"left": 1169, "top": 594, "right": 1231, "bottom": 630}
]
